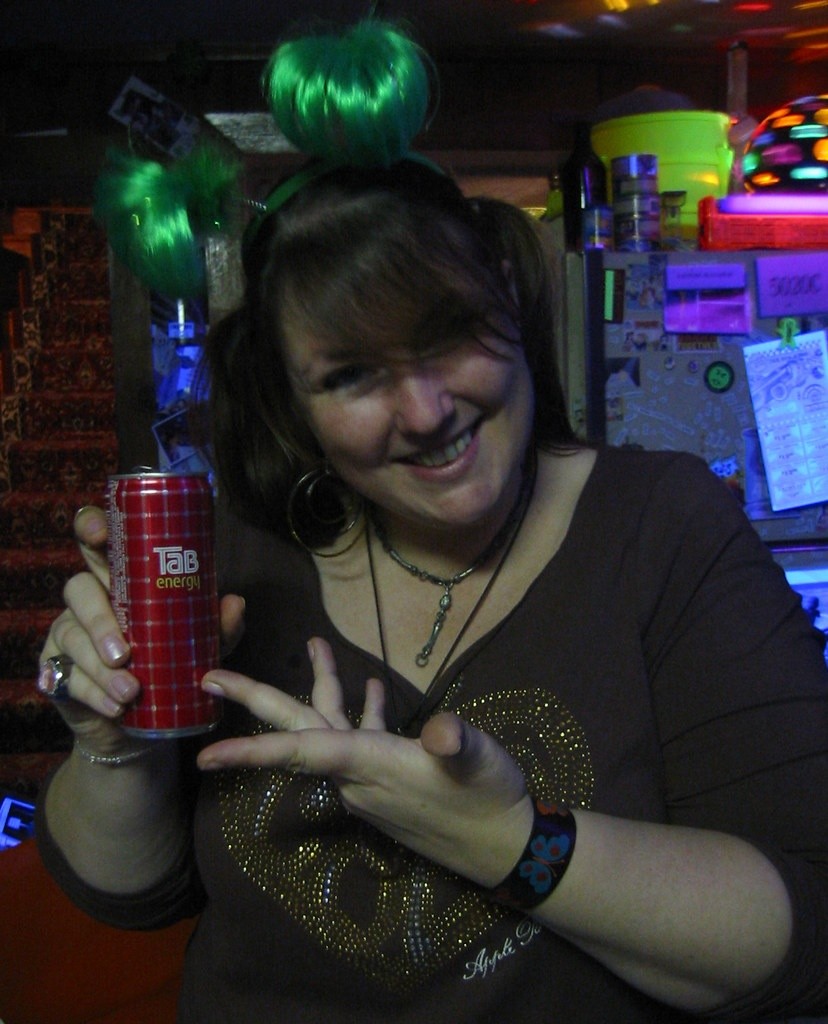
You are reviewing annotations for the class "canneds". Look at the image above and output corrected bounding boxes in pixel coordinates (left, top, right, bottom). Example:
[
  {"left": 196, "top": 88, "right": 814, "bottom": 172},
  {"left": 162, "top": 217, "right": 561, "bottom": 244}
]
[
  {"left": 581, "top": 153, "right": 665, "bottom": 255},
  {"left": 101, "top": 465, "right": 225, "bottom": 740}
]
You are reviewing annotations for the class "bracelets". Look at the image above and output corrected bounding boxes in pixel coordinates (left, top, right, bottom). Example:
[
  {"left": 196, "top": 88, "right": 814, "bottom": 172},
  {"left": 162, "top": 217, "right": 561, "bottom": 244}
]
[
  {"left": 75, "top": 741, "right": 165, "bottom": 764},
  {"left": 486, "top": 798, "right": 576, "bottom": 908}
]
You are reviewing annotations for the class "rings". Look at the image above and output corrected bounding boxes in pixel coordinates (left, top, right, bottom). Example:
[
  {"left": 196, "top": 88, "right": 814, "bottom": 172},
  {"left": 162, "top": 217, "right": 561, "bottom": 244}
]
[{"left": 37, "top": 654, "right": 72, "bottom": 696}]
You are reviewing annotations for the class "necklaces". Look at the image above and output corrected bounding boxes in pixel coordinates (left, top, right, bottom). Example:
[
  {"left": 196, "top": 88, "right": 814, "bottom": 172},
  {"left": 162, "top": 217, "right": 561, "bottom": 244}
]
[{"left": 371, "top": 452, "right": 533, "bottom": 666}]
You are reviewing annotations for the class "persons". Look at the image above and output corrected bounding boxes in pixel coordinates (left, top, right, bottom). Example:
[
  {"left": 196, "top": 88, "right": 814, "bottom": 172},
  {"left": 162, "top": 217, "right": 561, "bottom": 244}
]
[{"left": 35, "top": 166, "right": 828, "bottom": 1023}]
